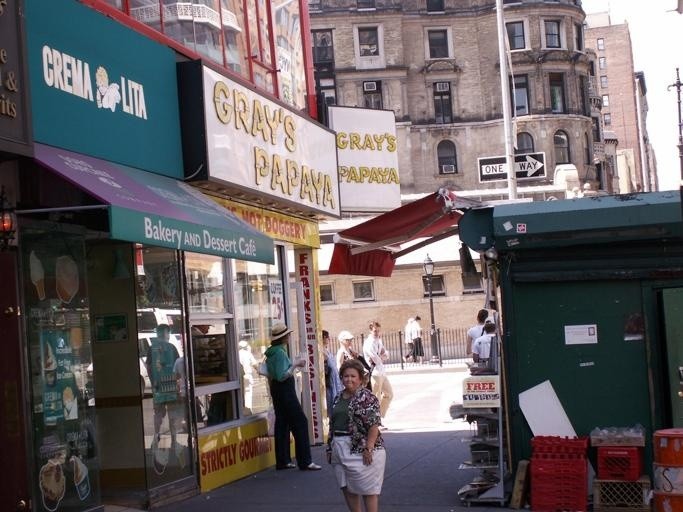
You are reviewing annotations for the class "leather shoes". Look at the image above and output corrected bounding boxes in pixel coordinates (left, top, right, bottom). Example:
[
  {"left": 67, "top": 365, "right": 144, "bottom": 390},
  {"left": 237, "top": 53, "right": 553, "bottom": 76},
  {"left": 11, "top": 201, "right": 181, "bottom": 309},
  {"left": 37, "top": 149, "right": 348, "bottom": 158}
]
[
  {"left": 277, "top": 460, "right": 297, "bottom": 469},
  {"left": 301, "top": 463, "right": 323, "bottom": 470}
]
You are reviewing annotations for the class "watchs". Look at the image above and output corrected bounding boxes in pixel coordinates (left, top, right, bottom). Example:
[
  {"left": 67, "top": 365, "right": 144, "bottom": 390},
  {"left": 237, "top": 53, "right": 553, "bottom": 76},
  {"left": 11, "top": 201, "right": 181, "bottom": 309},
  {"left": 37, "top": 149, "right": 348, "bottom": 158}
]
[{"left": 364, "top": 447, "right": 375, "bottom": 454}]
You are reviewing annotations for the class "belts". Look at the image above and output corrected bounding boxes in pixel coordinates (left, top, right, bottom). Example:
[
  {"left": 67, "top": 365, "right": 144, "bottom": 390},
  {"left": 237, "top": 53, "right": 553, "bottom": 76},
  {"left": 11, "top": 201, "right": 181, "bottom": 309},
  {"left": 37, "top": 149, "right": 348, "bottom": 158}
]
[{"left": 333, "top": 430, "right": 357, "bottom": 437}]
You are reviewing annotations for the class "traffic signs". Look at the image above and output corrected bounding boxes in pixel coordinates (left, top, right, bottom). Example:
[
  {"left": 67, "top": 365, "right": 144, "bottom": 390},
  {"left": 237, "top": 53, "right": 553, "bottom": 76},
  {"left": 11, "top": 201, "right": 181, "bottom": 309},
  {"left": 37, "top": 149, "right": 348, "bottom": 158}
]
[{"left": 476, "top": 149, "right": 547, "bottom": 186}]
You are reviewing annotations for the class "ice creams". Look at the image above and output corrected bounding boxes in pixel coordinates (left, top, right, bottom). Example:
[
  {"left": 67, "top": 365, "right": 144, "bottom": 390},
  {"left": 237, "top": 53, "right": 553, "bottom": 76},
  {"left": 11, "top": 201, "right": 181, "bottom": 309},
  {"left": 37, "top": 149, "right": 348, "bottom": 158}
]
[
  {"left": 73, "top": 455, "right": 91, "bottom": 501},
  {"left": 29, "top": 251, "right": 45, "bottom": 300},
  {"left": 55, "top": 255, "right": 79, "bottom": 305},
  {"left": 44, "top": 342, "right": 58, "bottom": 388},
  {"left": 40, "top": 458, "right": 65, "bottom": 512}
]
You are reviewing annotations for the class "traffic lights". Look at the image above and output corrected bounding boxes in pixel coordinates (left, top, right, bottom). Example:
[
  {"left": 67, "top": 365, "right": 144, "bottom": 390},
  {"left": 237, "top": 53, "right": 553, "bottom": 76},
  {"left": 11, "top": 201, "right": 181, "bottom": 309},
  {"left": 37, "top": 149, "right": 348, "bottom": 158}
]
[
  {"left": 257, "top": 279, "right": 263, "bottom": 291},
  {"left": 251, "top": 281, "right": 257, "bottom": 291}
]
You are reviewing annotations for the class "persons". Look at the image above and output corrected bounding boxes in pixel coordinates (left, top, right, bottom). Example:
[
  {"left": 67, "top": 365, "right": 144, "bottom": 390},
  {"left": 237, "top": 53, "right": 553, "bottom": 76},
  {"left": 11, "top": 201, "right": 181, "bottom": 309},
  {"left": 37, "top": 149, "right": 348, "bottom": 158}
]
[
  {"left": 335, "top": 330, "right": 358, "bottom": 372},
  {"left": 400, "top": 317, "right": 416, "bottom": 363},
  {"left": 470, "top": 315, "right": 495, "bottom": 365},
  {"left": 190, "top": 324, "right": 216, "bottom": 372},
  {"left": 410, "top": 315, "right": 424, "bottom": 365},
  {"left": 325, "top": 357, "right": 388, "bottom": 512},
  {"left": 363, "top": 321, "right": 394, "bottom": 432},
  {"left": 236, "top": 339, "right": 259, "bottom": 412},
  {"left": 171, "top": 355, "right": 189, "bottom": 435},
  {"left": 262, "top": 322, "right": 324, "bottom": 472},
  {"left": 321, "top": 329, "right": 345, "bottom": 420},
  {"left": 465, "top": 308, "right": 488, "bottom": 355},
  {"left": 142, "top": 323, "right": 181, "bottom": 452}
]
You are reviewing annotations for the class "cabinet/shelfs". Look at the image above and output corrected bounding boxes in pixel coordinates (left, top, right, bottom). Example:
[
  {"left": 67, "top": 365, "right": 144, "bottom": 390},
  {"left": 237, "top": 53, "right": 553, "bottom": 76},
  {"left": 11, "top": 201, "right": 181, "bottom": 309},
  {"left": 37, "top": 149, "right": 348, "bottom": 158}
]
[{"left": 452, "top": 311, "right": 516, "bottom": 509}]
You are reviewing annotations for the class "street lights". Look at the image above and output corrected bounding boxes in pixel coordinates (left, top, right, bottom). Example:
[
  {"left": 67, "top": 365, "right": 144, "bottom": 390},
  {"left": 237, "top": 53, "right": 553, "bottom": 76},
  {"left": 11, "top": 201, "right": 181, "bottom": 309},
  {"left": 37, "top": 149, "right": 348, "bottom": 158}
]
[{"left": 421, "top": 252, "right": 442, "bottom": 363}]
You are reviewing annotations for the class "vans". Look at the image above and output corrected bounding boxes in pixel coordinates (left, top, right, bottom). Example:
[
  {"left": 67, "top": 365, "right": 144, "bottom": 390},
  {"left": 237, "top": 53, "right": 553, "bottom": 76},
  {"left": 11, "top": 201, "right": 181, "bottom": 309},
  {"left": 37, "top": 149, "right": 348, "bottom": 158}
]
[{"left": 86, "top": 305, "right": 188, "bottom": 399}]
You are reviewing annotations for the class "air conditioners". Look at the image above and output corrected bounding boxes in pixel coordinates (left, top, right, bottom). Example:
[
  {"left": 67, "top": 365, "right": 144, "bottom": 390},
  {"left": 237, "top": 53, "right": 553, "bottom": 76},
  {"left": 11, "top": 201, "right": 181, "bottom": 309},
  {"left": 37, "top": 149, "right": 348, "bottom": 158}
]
[
  {"left": 362, "top": 81, "right": 377, "bottom": 92},
  {"left": 441, "top": 163, "right": 455, "bottom": 174},
  {"left": 436, "top": 82, "right": 449, "bottom": 92}
]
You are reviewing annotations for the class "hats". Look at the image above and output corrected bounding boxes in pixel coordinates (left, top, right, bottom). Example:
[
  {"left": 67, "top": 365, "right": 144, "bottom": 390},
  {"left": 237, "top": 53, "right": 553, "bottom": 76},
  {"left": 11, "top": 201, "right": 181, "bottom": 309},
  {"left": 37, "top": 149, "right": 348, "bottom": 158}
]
[
  {"left": 414, "top": 316, "right": 421, "bottom": 320},
  {"left": 270, "top": 321, "right": 293, "bottom": 341},
  {"left": 337, "top": 330, "right": 355, "bottom": 341}
]
